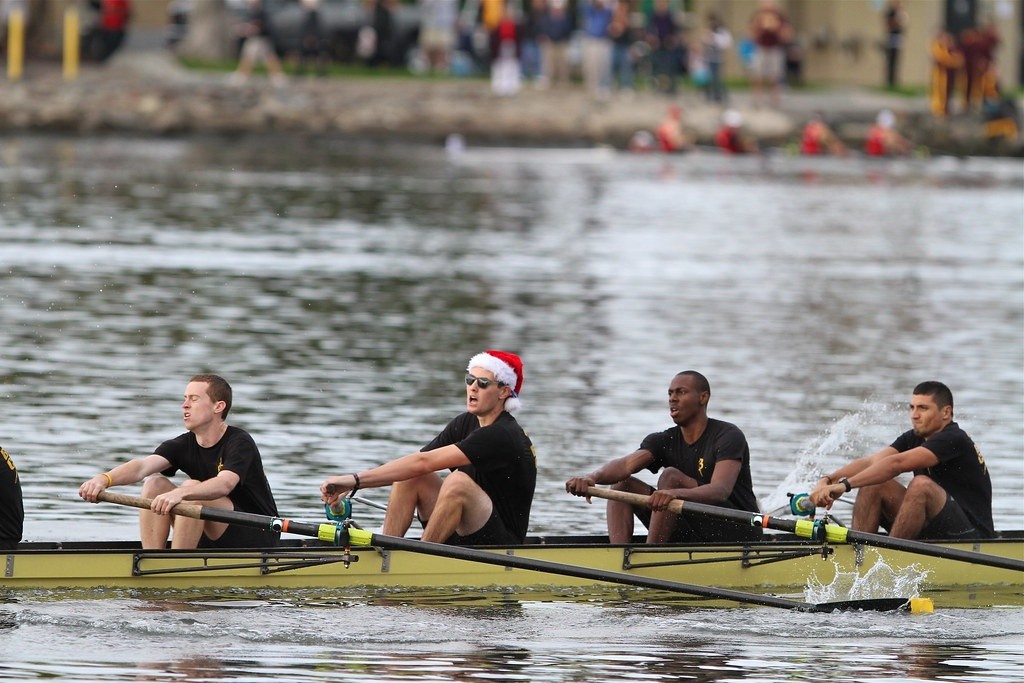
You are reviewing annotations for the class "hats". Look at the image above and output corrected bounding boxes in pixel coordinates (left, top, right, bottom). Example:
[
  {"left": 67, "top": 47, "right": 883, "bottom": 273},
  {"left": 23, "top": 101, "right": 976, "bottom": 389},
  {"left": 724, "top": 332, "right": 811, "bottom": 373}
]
[{"left": 466, "top": 350, "right": 523, "bottom": 398}]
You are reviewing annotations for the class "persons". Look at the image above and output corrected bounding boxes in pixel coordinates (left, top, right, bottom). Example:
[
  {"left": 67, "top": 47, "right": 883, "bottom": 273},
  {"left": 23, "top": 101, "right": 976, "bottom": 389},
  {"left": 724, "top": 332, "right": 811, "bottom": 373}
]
[
  {"left": 0, "top": 447, "right": 24, "bottom": 553},
  {"left": 321, "top": 349, "right": 537, "bottom": 548},
  {"left": 810, "top": 381, "right": 994, "bottom": 539},
  {"left": 77, "top": 375, "right": 280, "bottom": 550},
  {"left": 97, "top": 0, "right": 1024, "bottom": 172},
  {"left": 565, "top": 370, "right": 762, "bottom": 543}
]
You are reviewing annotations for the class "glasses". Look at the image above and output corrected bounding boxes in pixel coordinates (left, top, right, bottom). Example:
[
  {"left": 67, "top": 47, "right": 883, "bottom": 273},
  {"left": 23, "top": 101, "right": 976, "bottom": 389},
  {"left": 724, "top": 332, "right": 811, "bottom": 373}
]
[{"left": 465, "top": 373, "right": 501, "bottom": 390}]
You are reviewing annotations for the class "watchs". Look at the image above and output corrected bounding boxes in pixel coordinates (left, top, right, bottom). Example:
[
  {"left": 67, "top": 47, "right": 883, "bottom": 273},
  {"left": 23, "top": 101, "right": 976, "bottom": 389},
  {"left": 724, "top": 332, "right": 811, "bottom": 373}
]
[{"left": 838, "top": 476, "right": 851, "bottom": 492}]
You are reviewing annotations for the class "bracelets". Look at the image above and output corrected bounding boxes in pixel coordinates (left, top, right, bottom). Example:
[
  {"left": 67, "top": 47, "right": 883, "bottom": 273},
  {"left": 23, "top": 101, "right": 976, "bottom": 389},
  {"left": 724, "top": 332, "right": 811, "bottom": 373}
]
[
  {"left": 347, "top": 471, "right": 361, "bottom": 497},
  {"left": 101, "top": 473, "right": 112, "bottom": 488},
  {"left": 822, "top": 475, "right": 832, "bottom": 484}
]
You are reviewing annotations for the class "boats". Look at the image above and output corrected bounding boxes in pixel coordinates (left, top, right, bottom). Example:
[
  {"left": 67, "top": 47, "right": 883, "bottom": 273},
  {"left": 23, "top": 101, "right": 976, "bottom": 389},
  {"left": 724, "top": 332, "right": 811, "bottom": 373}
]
[{"left": 0, "top": 524, "right": 1024, "bottom": 591}]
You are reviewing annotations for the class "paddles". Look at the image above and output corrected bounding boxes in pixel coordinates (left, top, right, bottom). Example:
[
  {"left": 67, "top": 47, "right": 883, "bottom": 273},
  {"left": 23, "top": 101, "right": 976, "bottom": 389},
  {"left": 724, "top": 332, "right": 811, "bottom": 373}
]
[
  {"left": 587, "top": 486, "right": 1024, "bottom": 623},
  {"left": 95, "top": 489, "right": 936, "bottom": 616}
]
